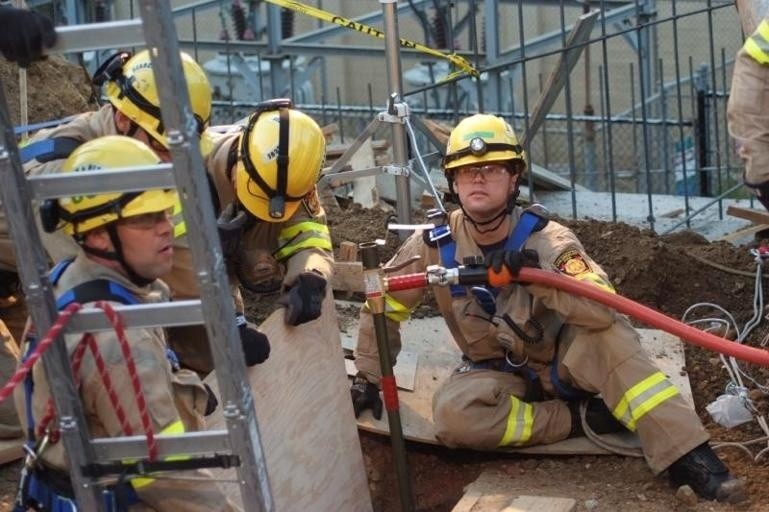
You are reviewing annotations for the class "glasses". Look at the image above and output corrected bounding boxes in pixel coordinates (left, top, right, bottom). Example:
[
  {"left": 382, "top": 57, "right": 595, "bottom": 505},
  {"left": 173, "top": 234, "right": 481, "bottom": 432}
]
[{"left": 454, "top": 164, "right": 509, "bottom": 180}]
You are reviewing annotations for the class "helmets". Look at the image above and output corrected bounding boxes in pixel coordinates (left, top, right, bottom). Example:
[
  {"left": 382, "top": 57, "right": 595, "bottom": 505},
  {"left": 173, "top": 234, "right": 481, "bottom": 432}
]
[
  {"left": 106, "top": 47, "right": 211, "bottom": 148},
  {"left": 445, "top": 114, "right": 527, "bottom": 171},
  {"left": 56, "top": 136, "right": 175, "bottom": 234},
  {"left": 236, "top": 107, "right": 325, "bottom": 222}
]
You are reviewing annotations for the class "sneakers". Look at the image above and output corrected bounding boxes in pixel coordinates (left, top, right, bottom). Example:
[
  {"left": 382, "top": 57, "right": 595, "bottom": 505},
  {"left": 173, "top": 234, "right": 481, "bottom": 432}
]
[
  {"left": 566, "top": 400, "right": 629, "bottom": 439},
  {"left": 670, "top": 442, "right": 746, "bottom": 503}
]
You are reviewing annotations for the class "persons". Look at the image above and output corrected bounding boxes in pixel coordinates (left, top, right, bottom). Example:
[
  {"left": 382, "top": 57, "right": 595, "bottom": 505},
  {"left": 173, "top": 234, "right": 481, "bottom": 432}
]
[
  {"left": 153, "top": 97, "right": 334, "bottom": 380},
  {"left": 1, "top": 49, "right": 245, "bottom": 512},
  {"left": 14, "top": 138, "right": 239, "bottom": 416},
  {"left": 725, "top": 0, "right": 769, "bottom": 213},
  {"left": 351, "top": 112, "right": 748, "bottom": 499}
]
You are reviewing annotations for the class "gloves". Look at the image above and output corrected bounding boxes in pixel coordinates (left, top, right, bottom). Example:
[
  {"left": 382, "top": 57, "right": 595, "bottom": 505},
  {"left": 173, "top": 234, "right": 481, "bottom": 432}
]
[
  {"left": 236, "top": 311, "right": 270, "bottom": 366},
  {"left": 349, "top": 373, "right": 382, "bottom": 420},
  {"left": 484, "top": 250, "right": 541, "bottom": 285},
  {"left": 273, "top": 272, "right": 326, "bottom": 324}
]
